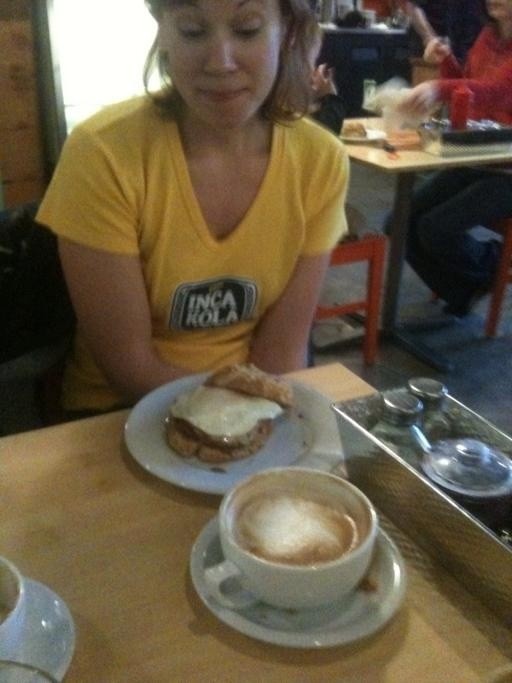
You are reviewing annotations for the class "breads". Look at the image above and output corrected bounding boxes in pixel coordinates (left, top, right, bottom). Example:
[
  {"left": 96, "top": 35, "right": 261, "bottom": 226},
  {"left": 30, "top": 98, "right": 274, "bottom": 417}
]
[
  {"left": 165, "top": 365, "right": 293, "bottom": 464},
  {"left": 342, "top": 122, "right": 367, "bottom": 138}
]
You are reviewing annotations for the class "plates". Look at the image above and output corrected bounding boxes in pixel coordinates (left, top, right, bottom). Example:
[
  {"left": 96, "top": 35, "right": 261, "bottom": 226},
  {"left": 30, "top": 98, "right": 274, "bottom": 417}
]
[
  {"left": 338, "top": 129, "right": 387, "bottom": 143},
  {"left": 123, "top": 369, "right": 344, "bottom": 496},
  {"left": 187, "top": 517, "right": 409, "bottom": 651},
  {"left": 1, "top": 575, "right": 79, "bottom": 683}
]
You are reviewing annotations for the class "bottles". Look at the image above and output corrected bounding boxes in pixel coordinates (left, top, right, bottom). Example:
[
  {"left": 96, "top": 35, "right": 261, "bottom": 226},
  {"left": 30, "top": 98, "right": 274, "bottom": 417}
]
[
  {"left": 407, "top": 377, "right": 451, "bottom": 442},
  {"left": 370, "top": 392, "right": 430, "bottom": 470}
]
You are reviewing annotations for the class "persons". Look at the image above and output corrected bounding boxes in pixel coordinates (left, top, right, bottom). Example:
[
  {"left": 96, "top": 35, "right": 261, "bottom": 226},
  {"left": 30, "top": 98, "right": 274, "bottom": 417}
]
[
  {"left": 34, "top": 1, "right": 350, "bottom": 423},
  {"left": 381, "top": 0, "right": 512, "bottom": 321},
  {"left": 311, "top": 63, "right": 414, "bottom": 138},
  {"left": 397, "top": 0, "right": 498, "bottom": 83}
]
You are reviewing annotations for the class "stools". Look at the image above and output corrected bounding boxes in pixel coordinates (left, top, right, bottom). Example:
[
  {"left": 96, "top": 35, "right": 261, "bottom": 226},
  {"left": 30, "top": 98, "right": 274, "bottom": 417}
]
[
  {"left": 483, "top": 215, "right": 512, "bottom": 340},
  {"left": 311, "top": 204, "right": 387, "bottom": 362}
]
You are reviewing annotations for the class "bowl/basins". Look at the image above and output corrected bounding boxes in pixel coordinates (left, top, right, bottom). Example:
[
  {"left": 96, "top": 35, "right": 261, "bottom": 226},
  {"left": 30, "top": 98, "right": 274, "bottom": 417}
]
[
  {"left": 1, "top": 555, "right": 26, "bottom": 660},
  {"left": 202, "top": 466, "right": 378, "bottom": 613}
]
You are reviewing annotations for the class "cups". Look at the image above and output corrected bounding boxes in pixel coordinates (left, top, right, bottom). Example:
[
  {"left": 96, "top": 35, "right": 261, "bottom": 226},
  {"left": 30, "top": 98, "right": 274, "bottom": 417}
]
[{"left": 448, "top": 89, "right": 473, "bottom": 130}]
